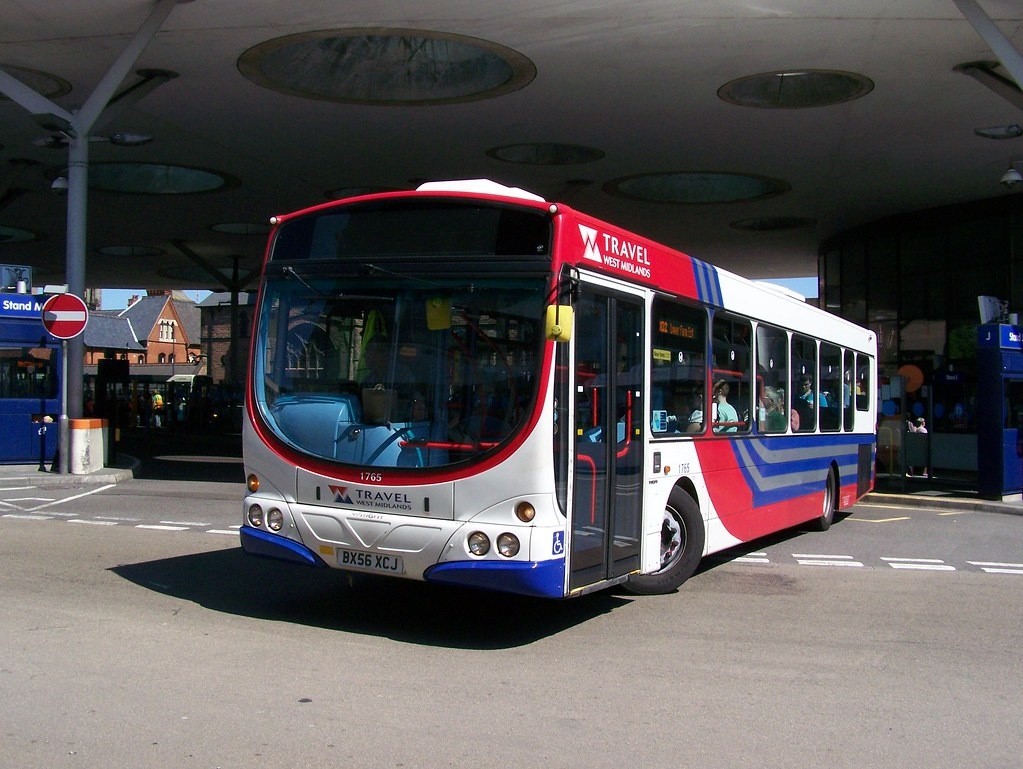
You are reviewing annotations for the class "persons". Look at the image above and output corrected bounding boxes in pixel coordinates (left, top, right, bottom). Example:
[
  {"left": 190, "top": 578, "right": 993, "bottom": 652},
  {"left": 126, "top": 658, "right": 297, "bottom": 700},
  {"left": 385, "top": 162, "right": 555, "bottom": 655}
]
[
  {"left": 905, "top": 412, "right": 914, "bottom": 476},
  {"left": 680, "top": 350, "right": 861, "bottom": 436},
  {"left": 914, "top": 416, "right": 930, "bottom": 477},
  {"left": 358, "top": 336, "right": 423, "bottom": 421}
]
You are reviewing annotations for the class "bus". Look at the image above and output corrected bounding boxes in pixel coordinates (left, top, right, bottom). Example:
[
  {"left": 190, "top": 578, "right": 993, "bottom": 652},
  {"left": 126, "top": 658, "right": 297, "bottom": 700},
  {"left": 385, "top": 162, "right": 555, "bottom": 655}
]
[{"left": 236, "top": 179, "right": 880, "bottom": 601}]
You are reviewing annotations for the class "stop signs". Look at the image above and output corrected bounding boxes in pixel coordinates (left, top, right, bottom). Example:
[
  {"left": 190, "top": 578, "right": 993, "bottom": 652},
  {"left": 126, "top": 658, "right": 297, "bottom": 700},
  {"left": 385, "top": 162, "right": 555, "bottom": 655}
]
[{"left": 42, "top": 294, "right": 89, "bottom": 339}]
